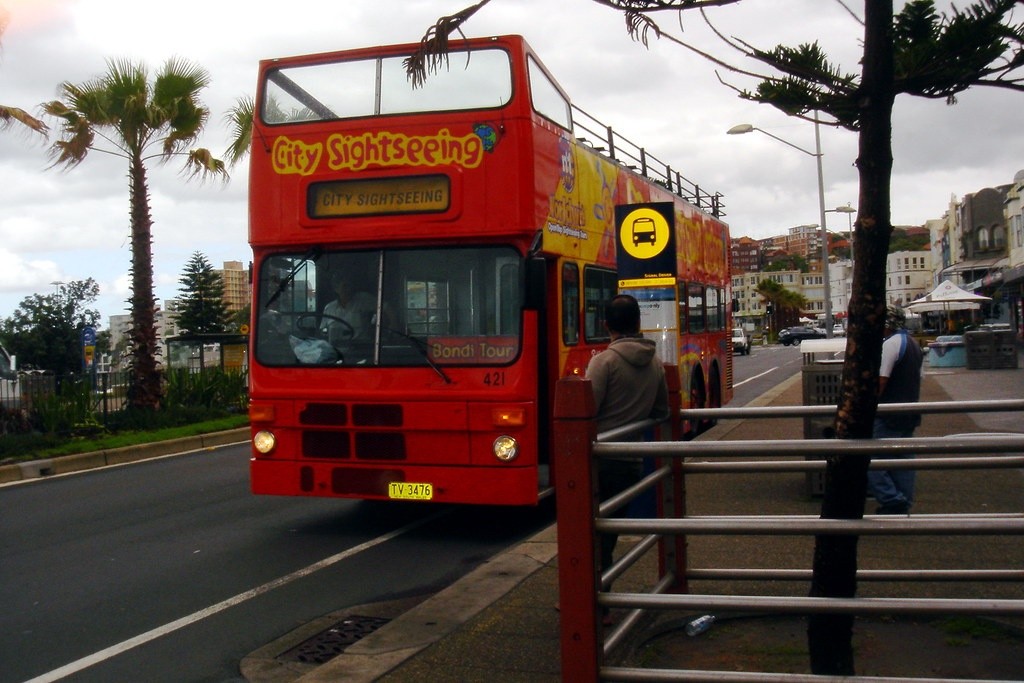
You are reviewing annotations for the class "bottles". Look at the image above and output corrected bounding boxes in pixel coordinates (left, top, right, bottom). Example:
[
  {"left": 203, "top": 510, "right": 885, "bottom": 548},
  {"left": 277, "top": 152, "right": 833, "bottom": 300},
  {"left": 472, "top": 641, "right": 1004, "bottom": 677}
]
[{"left": 687, "top": 615, "right": 715, "bottom": 636}]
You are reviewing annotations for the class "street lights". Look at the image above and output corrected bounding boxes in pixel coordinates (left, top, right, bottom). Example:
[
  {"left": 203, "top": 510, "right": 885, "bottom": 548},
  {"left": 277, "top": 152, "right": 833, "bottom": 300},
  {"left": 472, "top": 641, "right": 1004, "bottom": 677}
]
[
  {"left": 724, "top": 122, "right": 857, "bottom": 336},
  {"left": 802, "top": 223, "right": 854, "bottom": 277}
]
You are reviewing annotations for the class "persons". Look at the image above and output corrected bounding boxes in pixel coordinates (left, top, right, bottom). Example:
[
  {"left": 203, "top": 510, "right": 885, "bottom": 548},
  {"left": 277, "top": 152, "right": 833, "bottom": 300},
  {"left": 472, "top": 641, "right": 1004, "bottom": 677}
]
[
  {"left": 573, "top": 294, "right": 663, "bottom": 624},
  {"left": 856, "top": 302, "right": 924, "bottom": 515},
  {"left": 316, "top": 269, "right": 397, "bottom": 347}
]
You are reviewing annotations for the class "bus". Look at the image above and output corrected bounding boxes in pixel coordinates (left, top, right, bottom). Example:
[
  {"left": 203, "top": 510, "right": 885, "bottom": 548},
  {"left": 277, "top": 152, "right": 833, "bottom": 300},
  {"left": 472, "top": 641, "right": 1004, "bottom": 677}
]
[{"left": 244, "top": 32, "right": 737, "bottom": 506}]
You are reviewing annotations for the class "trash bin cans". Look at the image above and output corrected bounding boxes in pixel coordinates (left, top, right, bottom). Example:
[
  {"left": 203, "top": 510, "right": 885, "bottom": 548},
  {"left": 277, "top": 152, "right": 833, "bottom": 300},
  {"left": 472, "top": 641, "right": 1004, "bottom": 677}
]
[
  {"left": 964, "top": 323, "right": 1019, "bottom": 370},
  {"left": 798, "top": 334, "right": 848, "bottom": 445}
]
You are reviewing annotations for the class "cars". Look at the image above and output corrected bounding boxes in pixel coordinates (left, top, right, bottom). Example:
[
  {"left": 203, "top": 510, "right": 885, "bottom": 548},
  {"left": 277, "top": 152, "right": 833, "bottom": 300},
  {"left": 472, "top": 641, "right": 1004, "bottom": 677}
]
[
  {"left": 832, "top": 325, "right": 845, "bottom": 336},
  {"left": 777, "top": 326, "right": 827, "bottom": 347},
  {"left": 731, "top": 327, "right": 752, "bottom": 355}
]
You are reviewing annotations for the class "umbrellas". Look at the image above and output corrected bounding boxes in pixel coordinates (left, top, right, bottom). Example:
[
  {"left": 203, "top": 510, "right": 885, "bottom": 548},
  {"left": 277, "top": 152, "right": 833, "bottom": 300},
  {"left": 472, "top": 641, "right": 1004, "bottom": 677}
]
[{"left": 909, "top": 280, "right": 993, "bottom": 336}]
